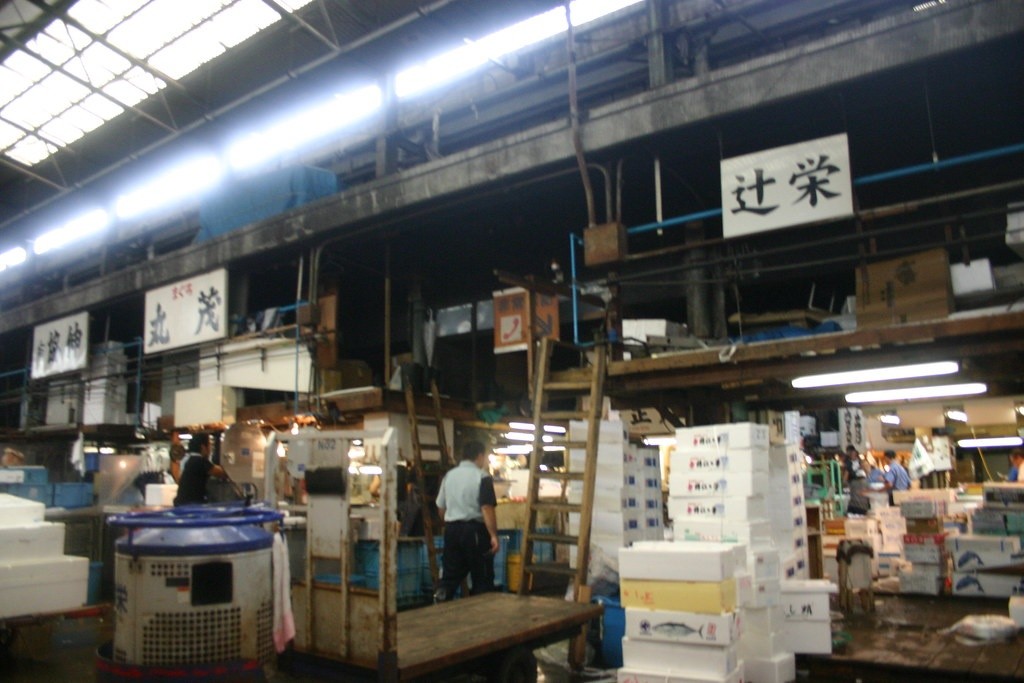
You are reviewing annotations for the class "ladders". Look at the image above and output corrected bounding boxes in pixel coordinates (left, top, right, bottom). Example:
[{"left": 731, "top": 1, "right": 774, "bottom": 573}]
[
  {"left": 402, "top": 374, "right": 471, "bottom": 600},
  {"left": 516, "top": 337, "right": 610, "bottom": 671}
]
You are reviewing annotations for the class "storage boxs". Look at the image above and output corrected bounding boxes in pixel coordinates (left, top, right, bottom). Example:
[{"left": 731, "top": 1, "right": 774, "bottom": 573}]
[{"left": 0, "top": 417, "right": 1024, "bottom": 683}]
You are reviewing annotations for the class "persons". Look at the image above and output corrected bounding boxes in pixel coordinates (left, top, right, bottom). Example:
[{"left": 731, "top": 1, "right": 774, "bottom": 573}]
[
  {"left": 999, "top": 448, "right": 1024, "bottom": 482},
  {"left": 233, "top": 312, "right": 263, "bottom": 336},
  {"left": 173, "top": 428, "right": 225, "bottom": 507},
  {"left": 434, "top": 439, "right": 500, "bottom": 605},
  {"left": 369, "top": 459, "right": 438, "bottom": 537},
  {"left": 809, "top": 445, "right": 911, "bottom": 516}
]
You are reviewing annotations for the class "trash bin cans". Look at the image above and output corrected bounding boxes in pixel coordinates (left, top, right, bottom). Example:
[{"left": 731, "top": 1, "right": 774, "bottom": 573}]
[{"left": 589, "top": 594, "right": 626, "bottom": 670}]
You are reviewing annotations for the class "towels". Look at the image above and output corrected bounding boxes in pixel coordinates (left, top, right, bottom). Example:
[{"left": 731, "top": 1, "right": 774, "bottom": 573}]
[{"left": 271, "top": 531, "right": 297, "bottom": 654}]
